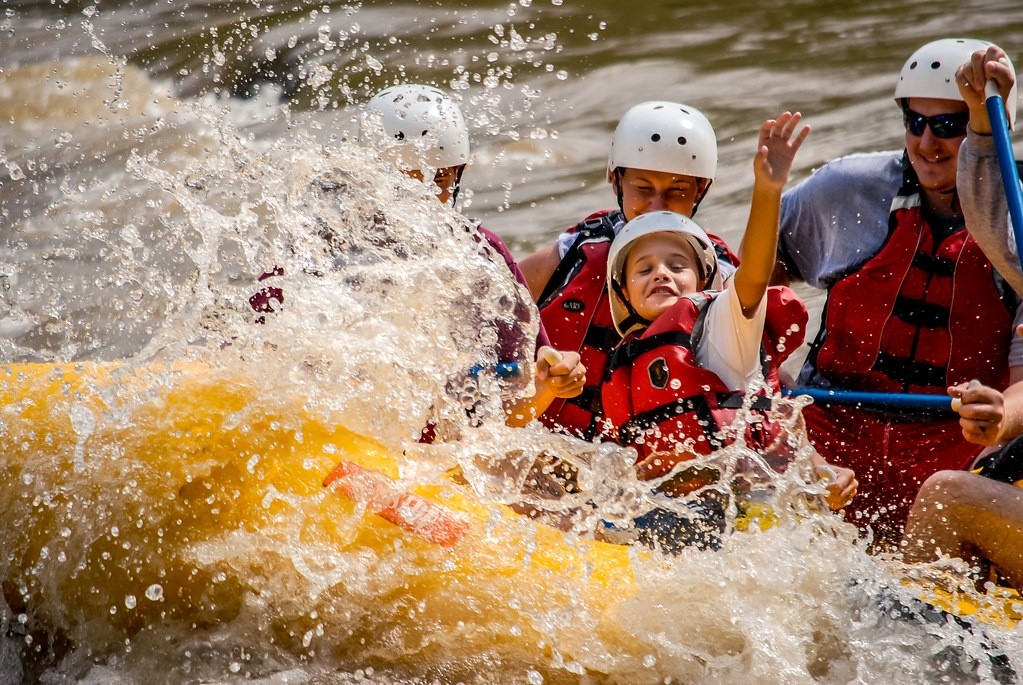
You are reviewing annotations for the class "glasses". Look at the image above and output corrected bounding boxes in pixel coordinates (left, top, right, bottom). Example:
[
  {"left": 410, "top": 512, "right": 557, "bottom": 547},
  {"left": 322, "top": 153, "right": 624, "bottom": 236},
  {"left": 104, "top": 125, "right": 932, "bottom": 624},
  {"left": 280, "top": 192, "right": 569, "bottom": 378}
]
[{"left": 903, "top": 106, "right": 970, "bottom": 138}]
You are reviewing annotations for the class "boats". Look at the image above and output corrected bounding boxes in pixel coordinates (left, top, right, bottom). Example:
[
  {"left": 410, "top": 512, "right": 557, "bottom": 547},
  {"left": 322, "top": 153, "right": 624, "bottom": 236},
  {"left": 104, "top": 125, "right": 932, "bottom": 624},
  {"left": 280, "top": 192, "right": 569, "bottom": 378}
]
[{"left": 0, "top": 356, "right": 838, "bottom": 685}]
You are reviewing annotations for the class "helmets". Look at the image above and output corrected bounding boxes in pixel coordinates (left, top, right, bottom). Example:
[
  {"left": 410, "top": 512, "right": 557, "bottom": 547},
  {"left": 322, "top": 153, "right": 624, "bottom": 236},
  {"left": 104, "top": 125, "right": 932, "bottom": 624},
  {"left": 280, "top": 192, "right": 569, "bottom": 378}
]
[
  {"left": 605, "top": 101, "right": 717, "bottom": 193},
  {"left": 607, "top": 210, "right": 725, "bottom": 338},
  {"left": 357, "top": 85, "right": 469, "bottom": 169},
  {"left": 893, "top": 39, "right": 1015, "bottom": 130}
]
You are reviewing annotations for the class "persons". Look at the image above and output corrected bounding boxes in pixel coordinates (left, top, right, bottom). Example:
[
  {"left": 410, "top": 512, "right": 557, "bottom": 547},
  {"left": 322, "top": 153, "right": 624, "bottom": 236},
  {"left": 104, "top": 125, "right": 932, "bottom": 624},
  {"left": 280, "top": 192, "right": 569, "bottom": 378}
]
[
  {"left": 516, "top": 101, "right": 859, "bottom": 510},
  {"left": 591, "top": 109, "right": 810, "bottom": 556},
  {"left": 901, "top": 47, "right": 1023, "bottom": 599},
  {"left": 248, "top": 83, "right": 586, "bottom": 484},
  {"left": 768, "top": 37, "right": 1023, "bottom": 548}
]
